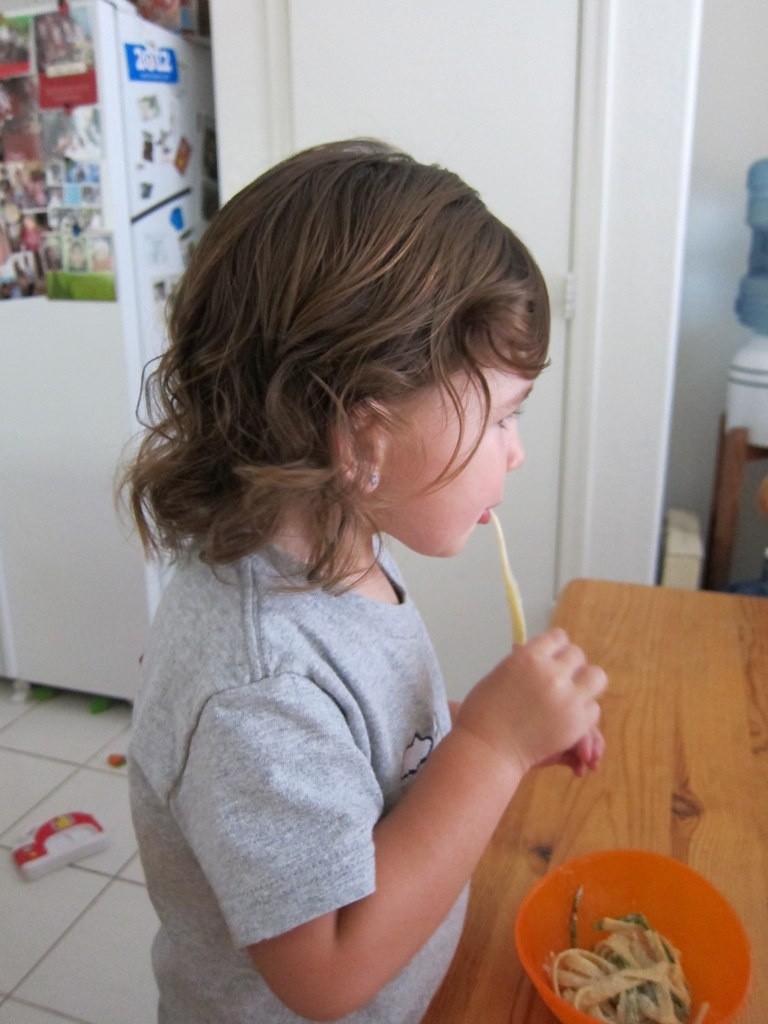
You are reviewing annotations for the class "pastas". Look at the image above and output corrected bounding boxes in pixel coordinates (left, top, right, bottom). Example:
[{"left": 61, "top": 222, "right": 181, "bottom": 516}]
[
  {"left": 552, "top": 917, "right": 708, "bottom": 1024},
  {"left": 489, "top": 509, "right": 527, "bottom": 646}
]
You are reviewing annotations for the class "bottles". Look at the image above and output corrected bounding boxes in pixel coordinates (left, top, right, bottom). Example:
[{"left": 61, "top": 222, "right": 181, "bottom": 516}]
[{"left": 734, "top": 158, "right": 768, "bottom": 333}]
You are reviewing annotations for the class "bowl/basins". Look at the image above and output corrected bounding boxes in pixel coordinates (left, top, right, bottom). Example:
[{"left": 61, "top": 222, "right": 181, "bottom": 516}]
[{"left": 514, "top": 849, "right": 752, "bottom": 1024}]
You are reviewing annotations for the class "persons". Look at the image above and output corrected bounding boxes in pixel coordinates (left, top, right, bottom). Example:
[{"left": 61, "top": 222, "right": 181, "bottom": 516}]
[
  {"left": 0, "top": 13, "right": 112, "bottom": 301},
  {"left": 112, "top": 136, "right": 612, "bottom": 1024}
]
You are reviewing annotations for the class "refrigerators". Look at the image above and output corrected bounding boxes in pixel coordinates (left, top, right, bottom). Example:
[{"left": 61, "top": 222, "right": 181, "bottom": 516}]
[{"left": 0, "top": 0, "right": 220, "bottom": 717}]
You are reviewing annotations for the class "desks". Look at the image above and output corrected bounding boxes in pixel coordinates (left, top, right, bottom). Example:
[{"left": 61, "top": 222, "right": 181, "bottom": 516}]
[{"left": 418, "top": 577, "right": 768, "bottom": 1024}]
